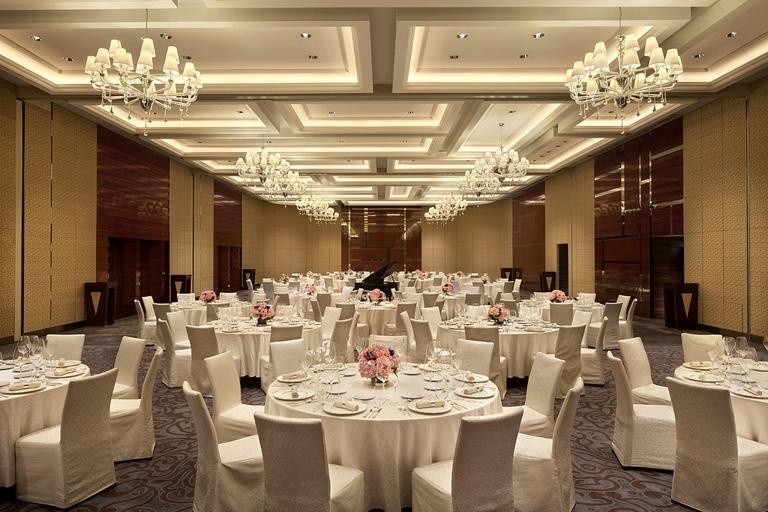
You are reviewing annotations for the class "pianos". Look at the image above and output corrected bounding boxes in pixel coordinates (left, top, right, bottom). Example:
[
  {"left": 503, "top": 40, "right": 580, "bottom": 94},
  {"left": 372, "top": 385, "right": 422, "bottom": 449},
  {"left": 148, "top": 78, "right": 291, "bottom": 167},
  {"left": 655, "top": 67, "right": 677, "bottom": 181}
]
[{"left": 354, "top": 261, "right": 400, "bottom": 301}]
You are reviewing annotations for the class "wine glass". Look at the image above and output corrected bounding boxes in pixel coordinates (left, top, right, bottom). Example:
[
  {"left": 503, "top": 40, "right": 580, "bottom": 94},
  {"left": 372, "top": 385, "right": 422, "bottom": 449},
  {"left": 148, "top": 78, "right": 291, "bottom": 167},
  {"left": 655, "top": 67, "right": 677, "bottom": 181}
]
[
  {"left": 562, "top": 294, "right": 600, "bottom": 308},
  {"left": 173, "top": 295, "right": 249, "bottom": 308},
  {"left": 205, "top": 307, "right": 257, "bottom": 334},
  {"left": 13, "top": 335, "right": 53, "bottom": 381},
  {"left": 763, "top": 333, "right": 768, "bottom": 351},
  {"left": 342, "top": 295, "right": 406, "bottom": 310},
  {"left": 707, "top": 336, "right": 758, "bottom": 389},
  {"left": 441, "top": 305, "right": 490, "bottom": 331},
  {"left": 513, "top": 300, "right": 560, "bottom": 333},
  {"left": 423, "top": 338, "right": 456, "bottom": 399},
  {"left": 305, "top": 339, "right": 340, "bottom": 405},
  {"left": 275, "top": 301, "right": 322, "bottom": 329}
]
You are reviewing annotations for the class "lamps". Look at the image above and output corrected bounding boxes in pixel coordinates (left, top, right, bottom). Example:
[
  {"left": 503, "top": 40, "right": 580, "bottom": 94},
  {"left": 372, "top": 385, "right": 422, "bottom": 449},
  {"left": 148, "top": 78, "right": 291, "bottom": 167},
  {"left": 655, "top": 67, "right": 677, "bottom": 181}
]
[
  {"left": 82, "top": 6, "right": 201, "bottom": 141},
  {"left": 238, "top": 145, "right": 341, "bottom": 224},
  {"left": 419, "top": 125, "right": 528, "bottom": 228},
  {"left": 564, "top": 5, "right": 684, "bottom": 136}
]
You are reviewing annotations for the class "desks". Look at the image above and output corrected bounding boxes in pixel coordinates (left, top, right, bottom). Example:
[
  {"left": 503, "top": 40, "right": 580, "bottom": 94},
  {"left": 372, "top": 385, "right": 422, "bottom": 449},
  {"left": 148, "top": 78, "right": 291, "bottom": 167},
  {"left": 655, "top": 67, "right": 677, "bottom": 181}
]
[
  {"left": 267, "top": 359, "right": 501, "bottom": 512},
  {"left": 673, "top": 357, "right": 768, "bottom": 444}
]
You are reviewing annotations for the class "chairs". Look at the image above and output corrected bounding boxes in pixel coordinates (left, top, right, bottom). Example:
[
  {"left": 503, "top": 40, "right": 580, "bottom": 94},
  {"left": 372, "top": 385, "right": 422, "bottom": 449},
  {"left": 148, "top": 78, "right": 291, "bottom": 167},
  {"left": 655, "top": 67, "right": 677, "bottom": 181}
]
[
  {"left": 16, "top": 369, "right": 121, "bottom": 510},
  {"left": 405, "top": 410, "right": 526, "bottom": 511},
  {"left": 180, "top": 379, "right": 263, "bottom": 511},
  {"left": 664, "top": 374, "right": 763, "bottom": 511},
  {"left": 202, "top": 350, "right": 264, "bottom": 442},
  {"left": 615, "top": 337, "right": 672, "bottom": 404},
  {"left": 268, "top": 338, "right": 308, "bottom": 376},
  {"left": 130, "top": 273, "right": 639, "bottom": 397},
  {"left": 45, "top": 333, "right": 87, "bottom": 363},
  {"left": 367, "top": 333, "right": 408, "bottom": 363},
  {"left": 679, "top": 331, "right": 726, "bottom": 361},
  {"left": 110, "top": 346, "right": 166, "bottom": 460},
  {"left": 516, "top": 374, "right": 584, "bottom": 512},
  {"left": 502, "top": 349, "right": 567, "bottom": 437},
  {"left": 455, "top": 337, "right": 494, "bottom": 379},
  {"left": 244, "top": 412, "right": 366, "bottom": 511},
  {"left": 604, "top": 350, "right": 676, "bottom": 472},
  {"left": 112, "top": 336, "right": 146, "bottom": 398}
]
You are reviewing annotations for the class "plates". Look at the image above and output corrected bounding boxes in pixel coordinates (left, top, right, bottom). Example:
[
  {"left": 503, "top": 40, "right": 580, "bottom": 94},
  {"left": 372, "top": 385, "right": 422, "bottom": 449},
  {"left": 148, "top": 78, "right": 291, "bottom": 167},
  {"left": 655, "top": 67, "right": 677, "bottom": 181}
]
[
  {"left": 274, "top": 364, "right": 384, "bottom": 419},
  {"left": 0, "top": 356, "right": 87, "bottom": 394},
  {"left": 682, "top": 360, "right": 768, "bottom": 400},
  {"left": 403, "top": 362, "right": 496, "bottom": 414}
]
